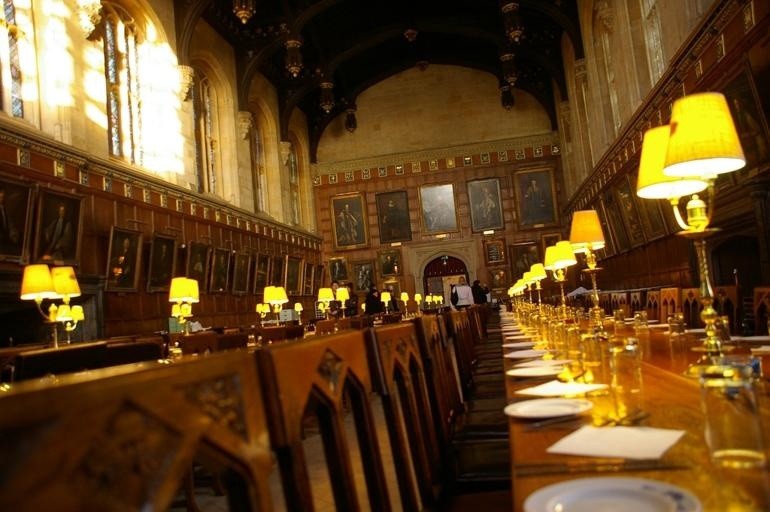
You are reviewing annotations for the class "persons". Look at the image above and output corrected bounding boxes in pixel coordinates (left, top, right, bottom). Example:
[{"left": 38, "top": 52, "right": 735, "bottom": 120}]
[
  {"left": 451, "top": 274, "right": 475, "bottom": 309},
  {"left": 386, "top": 286, "right": 399, "bottom": 311},
  {"left": 478, "top": 187, "right": 497, "bottom": 223},
  {"left": 328, "top": 280, "right": 341, "bottom": 317},
  {"left": 344, "top": 281, "right": 359, "bottom": 314},
  {"left": 333, "top": 261, "right": 345, "bottom": 279},
  {"left": 366, "top": 288, "right": 384, "bottom": 315},
  {"left": 523, "top": 177, "right": 546, "bottom": 221},
  {"left": 358, "top": 263, "right": 369, "bottom": 289},
  {"left": 470, "top": 279, "right": 489, "bottom": 305},
  {"left": 382, "top": 254, "right": 396, "bottom": 274},
  {"left": 426, "top": 194, "right": 451, "bottom": 230},
  {"left": 336, "top": 203, "right": 360, "bottom": 241},
  {"left": 367, "top": 281, "right": 381, "bottom": 302},
  {"left": 381, "top": 200, "right": 407, "bottom": 240}
]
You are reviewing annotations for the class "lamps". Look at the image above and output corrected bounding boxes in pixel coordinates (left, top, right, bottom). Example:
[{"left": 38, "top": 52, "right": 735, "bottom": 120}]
[
  {"left": 507, "top": 239, "right": 578, "bottom": 321},
  {"left": 19, "top": 264, "right": 86, "bottom": 350},
  {"left": 635, "top": 91, "right": 749, "bottom": 354},
  {"left": 568, "top": 210, "right": 610, "bottom": 340},
  {"left": 167, "top": 275, "right": 200, "bottom": 334}
]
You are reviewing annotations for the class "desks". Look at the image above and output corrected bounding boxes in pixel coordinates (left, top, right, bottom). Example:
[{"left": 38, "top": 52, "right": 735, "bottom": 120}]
[{"left": 498, "top": 300, "right": 770, "bottom": 511}]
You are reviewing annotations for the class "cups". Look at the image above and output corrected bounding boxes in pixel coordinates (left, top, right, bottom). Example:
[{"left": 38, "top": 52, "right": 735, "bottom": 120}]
[
  {"left": 567, "top": 306, "right": 734, "bottom": 353},
  {"left": 611, "top": 345, "right": 645, "bottom": 394},
  {"left": 696, "top": 360, "right": 769, "bottom": 469}
]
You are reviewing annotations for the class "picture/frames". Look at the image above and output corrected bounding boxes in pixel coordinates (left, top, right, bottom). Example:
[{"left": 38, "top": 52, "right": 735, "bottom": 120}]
[
  {"left": 577, "top": 62, "right": 770, "bottom": 272},
  {"left": 0, "top": 172, "right": 38, "bottom": 264},
  {"left": 146, "top": 230, "right": 178, "bottom": 295},
  {"left": 185, "top": 239, "right": 325, "bottom": 298},
  {"left": 466, "top": 176, "right": 506, "bottom": 234},
  {"left": 327, "top": 188, "right": 414, "bottom": 293},
  {"left": 103, "top": 226, "right": 145, "bottom": 294},
  {"left": 511, "top": 165, "right": 562, "bottom": 233},
  {"left": 33, "top": 184, "right": 88, "bottom": 267},
  {"left": 416, "top": 180, "right": 462, "bottom": 237}
]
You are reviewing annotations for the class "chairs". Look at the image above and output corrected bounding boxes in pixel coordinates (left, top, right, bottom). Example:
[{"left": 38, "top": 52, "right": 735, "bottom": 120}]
[
  {"left": 531, "top": 281, "right": 770, "bottom": 346},
  {"left": 0, "top": 286, "right": 511, "bottom": 510}
]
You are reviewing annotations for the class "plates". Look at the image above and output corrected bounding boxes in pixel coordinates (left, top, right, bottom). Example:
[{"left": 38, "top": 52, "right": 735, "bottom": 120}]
[
  {"left": 523, "top": 474, "right": 699, "bottom": 511},
  {"left": 498, "top": 308, "right": 574, "bottom": 378},
  {"left": 503, "top": 397, "right": 585, "bottom": 421}
]
